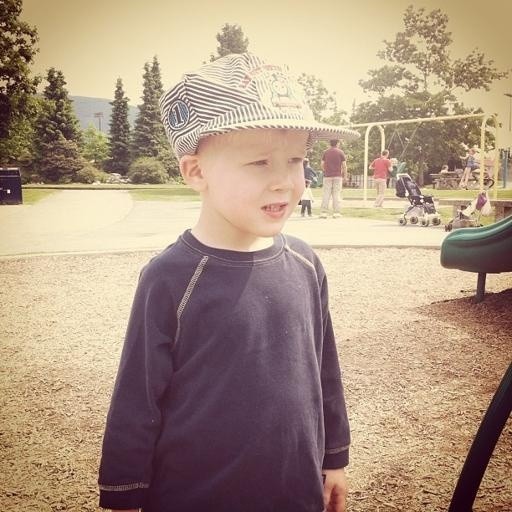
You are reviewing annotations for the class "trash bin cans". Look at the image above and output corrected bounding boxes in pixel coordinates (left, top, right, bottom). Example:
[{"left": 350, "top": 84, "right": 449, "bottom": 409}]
[{"left": 0, "top": 167, "right": 23, "bottom": 204}]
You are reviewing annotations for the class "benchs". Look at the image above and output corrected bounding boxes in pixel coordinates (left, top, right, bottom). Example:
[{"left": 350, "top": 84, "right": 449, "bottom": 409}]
[{"left": 439, "top": 199, "right": 512, "bottom": 229}]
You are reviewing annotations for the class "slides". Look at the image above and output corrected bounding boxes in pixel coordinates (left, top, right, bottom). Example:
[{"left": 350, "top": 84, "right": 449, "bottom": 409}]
[{"left": 441, "top": 215, "right": 512, "bottom": 302}]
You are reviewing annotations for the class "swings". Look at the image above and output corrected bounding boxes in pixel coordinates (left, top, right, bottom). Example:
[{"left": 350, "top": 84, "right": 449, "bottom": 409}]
[
  {"left": 310, "top": 126, "right": 359, "bottom": 189},
  {"left": 387, "top": 122, "right": 422, "bottom": 177},
  {"left": 439, "top": 118, "right": 480, "bottom": 172}
]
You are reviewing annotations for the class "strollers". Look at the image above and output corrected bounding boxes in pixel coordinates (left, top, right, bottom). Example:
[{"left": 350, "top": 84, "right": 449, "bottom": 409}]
[
  {"left": 395, "top": 173, "right": 441, "bottom": 227},
  {"left": 444, "top": 178, "right": 495, "bottom": 232}
]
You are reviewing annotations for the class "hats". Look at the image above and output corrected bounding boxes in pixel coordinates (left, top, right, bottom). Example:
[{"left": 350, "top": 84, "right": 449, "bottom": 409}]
[{"left": 155, "top": 50, "right": 362, "bottom": 162}]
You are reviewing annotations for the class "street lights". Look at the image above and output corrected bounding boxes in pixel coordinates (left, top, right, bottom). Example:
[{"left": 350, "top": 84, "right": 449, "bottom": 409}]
[{"left": 503, "top": 93, "right": 512, "bottom": 132}]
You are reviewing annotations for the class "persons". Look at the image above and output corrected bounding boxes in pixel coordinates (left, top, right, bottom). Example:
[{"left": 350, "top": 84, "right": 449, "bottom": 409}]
[
  {"left": 300, "top": 179, "right": 312, "bottom": 217},
  {"left": 369, "top": 149, "right": 394, "bottom": 207},
  {"left": 299, "top": 158, "right": 318, "bottom": 205},
  {"left": 459, "top": 148, "right": 476, "bottom": 186},
  {"left": 318, "top": 139, "right": 347, "bottom": 218},
  {"left": 97, "top": 53, "right": 351, "bottom": 512}
]
